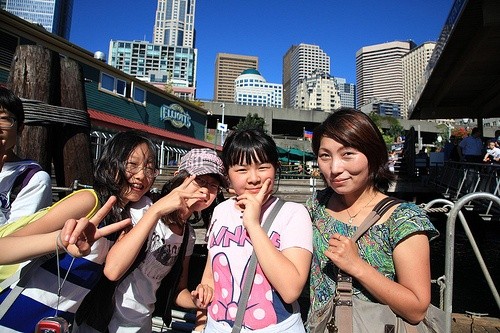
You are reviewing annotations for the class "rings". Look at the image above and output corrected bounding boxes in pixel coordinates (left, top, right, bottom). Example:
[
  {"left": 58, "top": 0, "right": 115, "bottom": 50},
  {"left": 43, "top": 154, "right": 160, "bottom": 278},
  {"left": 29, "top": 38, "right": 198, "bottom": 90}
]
[{"left": 337, "top": 235, "right": 342, "bottom": 239}]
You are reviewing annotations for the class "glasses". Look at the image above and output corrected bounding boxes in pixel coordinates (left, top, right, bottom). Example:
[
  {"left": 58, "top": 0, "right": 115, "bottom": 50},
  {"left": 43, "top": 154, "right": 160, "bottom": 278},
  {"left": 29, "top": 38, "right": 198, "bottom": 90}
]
[
  {"left": 0, "top": 115, "right": 18, "bottom": 128},
  {"left": 123, "top": 159, "right": 159, "bottom": 178}
]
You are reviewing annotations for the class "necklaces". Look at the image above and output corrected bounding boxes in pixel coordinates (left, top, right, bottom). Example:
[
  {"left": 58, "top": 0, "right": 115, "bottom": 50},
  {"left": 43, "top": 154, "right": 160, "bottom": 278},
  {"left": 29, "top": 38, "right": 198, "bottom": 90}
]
[{"left": 342, "top": 190, "right": 378, "bottom": 225}]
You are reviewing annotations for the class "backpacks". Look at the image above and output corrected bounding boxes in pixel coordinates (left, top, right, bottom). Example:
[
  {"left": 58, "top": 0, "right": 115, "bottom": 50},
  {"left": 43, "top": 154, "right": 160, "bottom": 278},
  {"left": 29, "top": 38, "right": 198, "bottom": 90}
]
[{"left": 0, "top": 189, "right": 102, "bottom": 281}]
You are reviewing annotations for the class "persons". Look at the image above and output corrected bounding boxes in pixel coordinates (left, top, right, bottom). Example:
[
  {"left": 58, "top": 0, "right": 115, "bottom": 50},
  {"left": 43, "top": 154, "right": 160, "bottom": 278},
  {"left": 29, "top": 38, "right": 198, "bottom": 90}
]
[{"left": 0, "top": 87, "right": 500, "bottom": 333}]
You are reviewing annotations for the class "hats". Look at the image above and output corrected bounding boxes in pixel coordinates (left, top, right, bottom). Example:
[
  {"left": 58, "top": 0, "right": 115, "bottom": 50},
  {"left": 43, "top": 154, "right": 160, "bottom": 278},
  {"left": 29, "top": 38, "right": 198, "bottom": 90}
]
[{"left": 178, "top": 148, "right": 224, "bottom": 177}]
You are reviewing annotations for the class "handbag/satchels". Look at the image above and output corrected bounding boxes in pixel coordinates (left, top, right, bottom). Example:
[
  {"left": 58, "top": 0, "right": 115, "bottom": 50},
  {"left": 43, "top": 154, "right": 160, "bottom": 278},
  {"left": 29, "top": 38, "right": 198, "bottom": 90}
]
[{"left": 309, "top": 197, "right": 461, "bottom": 333}]
